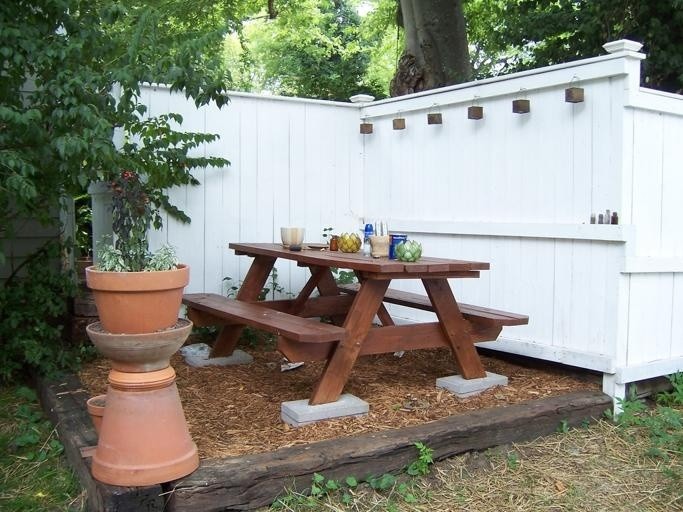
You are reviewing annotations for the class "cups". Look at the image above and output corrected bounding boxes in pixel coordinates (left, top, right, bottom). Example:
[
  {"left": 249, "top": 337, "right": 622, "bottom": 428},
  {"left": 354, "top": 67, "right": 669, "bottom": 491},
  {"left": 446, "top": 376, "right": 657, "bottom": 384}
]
[{"left": 388, "top": 234, "right": 411, "bottom": 260}]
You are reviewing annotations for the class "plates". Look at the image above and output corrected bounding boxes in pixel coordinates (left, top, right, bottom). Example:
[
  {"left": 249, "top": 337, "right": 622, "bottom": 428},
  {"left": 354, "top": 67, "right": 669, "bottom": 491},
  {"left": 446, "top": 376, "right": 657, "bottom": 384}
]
[{"left": 308, "top": 245, "right": 328, "bottom": 249}]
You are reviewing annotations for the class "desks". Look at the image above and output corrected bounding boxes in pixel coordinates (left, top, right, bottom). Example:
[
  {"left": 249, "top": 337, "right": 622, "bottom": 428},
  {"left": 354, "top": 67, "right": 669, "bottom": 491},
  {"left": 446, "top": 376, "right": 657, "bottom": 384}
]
[{"left": 208, "top": 239, "right": 491, "bottom": 407}]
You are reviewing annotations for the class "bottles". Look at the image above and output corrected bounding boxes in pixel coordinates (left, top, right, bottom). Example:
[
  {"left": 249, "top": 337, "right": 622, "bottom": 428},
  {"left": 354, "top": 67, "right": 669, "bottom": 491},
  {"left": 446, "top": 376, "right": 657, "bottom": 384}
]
[
  {"left": 364, "top": 224, "right": 374, "bottom": 245},
  {"left": 603, "top": 209, "right": 611, "bottom": 224},
  {"left": 329, "top": 235, "right": 338, "bottom": 251},
  {"left": 611, "top": 212, "right": 618, "bottom": 225}
]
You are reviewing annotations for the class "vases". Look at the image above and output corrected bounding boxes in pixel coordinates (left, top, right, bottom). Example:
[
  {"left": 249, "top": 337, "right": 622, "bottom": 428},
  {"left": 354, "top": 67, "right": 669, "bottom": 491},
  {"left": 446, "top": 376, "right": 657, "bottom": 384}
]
[{"left": 84, "top": 319, "right": 201, "bottom": 488}]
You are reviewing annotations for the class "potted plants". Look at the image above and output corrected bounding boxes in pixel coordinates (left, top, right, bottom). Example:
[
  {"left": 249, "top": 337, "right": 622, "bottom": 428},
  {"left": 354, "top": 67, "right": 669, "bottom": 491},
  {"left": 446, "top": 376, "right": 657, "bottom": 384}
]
[{"left": 86, "top": 107, "right": 230, "bottom": 334}]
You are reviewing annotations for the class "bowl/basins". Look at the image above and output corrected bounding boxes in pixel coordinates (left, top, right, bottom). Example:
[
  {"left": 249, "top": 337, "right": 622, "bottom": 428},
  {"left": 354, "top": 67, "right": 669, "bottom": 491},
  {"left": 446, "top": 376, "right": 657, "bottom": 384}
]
[{"left": 280, "top": 227, "right": 306, "bottom": 246}]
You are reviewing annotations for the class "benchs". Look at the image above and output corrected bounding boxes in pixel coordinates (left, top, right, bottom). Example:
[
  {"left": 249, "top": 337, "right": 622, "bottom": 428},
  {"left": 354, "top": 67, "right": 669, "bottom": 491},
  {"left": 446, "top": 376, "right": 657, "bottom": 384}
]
[
  {"left": 182, "top": 291, "right": 340, "bottom": 346},
  {"left": 341, "top": 280, "right": 529, "bottom": 332}
]
